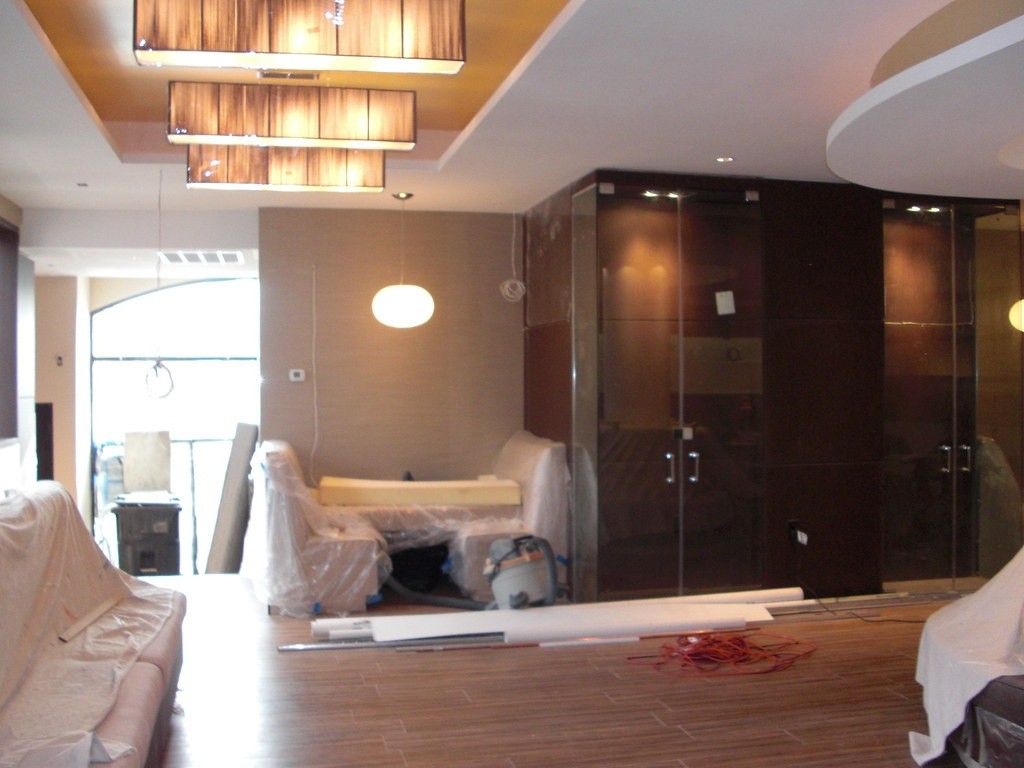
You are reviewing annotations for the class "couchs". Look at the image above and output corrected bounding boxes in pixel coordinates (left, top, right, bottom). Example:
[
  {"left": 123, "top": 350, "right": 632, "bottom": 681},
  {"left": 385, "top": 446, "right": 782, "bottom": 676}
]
[
  {"left": 0, "top": 479, "right": 188, "bottom": 768},
  {"left": 247, "top": 437, "right": 394, "bottom": 620},
  {"left": 443, "top": 428, "right": 576, "bottom": 607}
]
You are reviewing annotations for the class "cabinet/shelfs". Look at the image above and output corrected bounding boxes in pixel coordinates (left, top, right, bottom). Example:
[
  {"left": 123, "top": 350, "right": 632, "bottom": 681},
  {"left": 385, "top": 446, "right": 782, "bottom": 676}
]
[{"left": 511, "top": 165, "right": 982, "bottom": 599}]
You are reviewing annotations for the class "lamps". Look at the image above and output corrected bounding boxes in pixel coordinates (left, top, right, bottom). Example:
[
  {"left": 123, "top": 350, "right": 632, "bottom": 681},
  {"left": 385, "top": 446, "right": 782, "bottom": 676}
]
[
  {"left": 132, "top": 0, "right": 467, "bottom": 194},
  {"left": 372, "top": 189, "right": 434, "bottom": 330}
]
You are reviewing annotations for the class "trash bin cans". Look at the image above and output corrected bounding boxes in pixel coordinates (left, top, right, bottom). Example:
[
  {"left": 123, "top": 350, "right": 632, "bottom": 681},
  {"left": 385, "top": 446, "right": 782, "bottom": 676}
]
[{"left": 112, "top": 490, "right": 183, "bottom": 578}]
